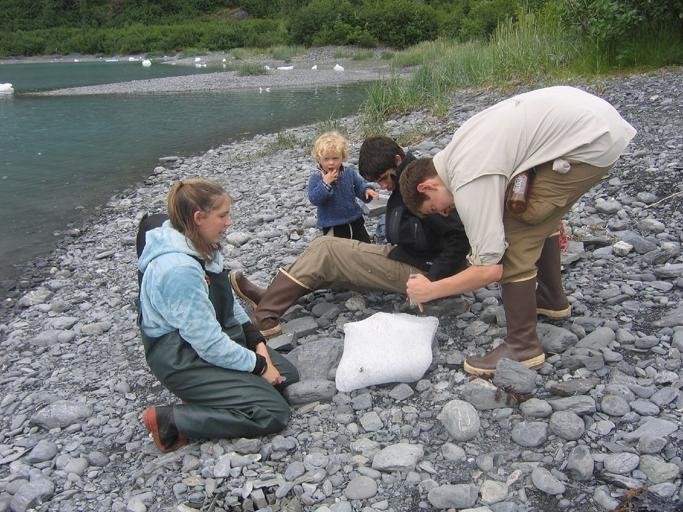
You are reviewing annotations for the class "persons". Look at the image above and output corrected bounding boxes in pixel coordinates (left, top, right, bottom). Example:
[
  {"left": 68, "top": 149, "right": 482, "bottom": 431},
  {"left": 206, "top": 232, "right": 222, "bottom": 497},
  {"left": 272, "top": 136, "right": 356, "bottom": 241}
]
[
  {"left": 136, "top": 177, "right": 299, "bottom": 454},
  {"left": 307, "top": 130, "right": 379, "bottom": 244},
  {"left": 398, "top": 85, "right": 637, "bottom": 378},
  {"left": 229, "top": 137, "right": 471, "bottom": 339}
]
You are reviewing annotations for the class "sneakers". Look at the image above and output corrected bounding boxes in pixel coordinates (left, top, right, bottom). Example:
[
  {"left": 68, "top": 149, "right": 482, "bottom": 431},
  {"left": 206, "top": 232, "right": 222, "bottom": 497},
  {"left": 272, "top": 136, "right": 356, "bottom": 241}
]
[{"left": 230, "top": 271, "right": 267, "bottom": 310}]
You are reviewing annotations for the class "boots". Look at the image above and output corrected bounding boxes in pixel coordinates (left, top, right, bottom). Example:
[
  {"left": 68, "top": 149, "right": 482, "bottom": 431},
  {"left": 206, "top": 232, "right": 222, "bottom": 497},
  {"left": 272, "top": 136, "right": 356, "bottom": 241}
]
[
  {"left": 463, "top": 273, "right": 545, "bottom": 377},
  {"left": 142, "top": 404, "right": 186, "bottom": 454},
  {"left": 535, "top": 231, "right": 572, "bottom": 320},
  {"left": 250, "top": 266, "right": 315, "bottom": 337}
]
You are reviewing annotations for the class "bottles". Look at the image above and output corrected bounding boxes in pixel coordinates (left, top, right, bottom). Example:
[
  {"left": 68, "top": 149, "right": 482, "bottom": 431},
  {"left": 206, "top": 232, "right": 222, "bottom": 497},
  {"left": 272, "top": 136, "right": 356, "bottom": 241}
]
[{"left": 505, "top": 170, "right": 532, "bottom": 213}]
